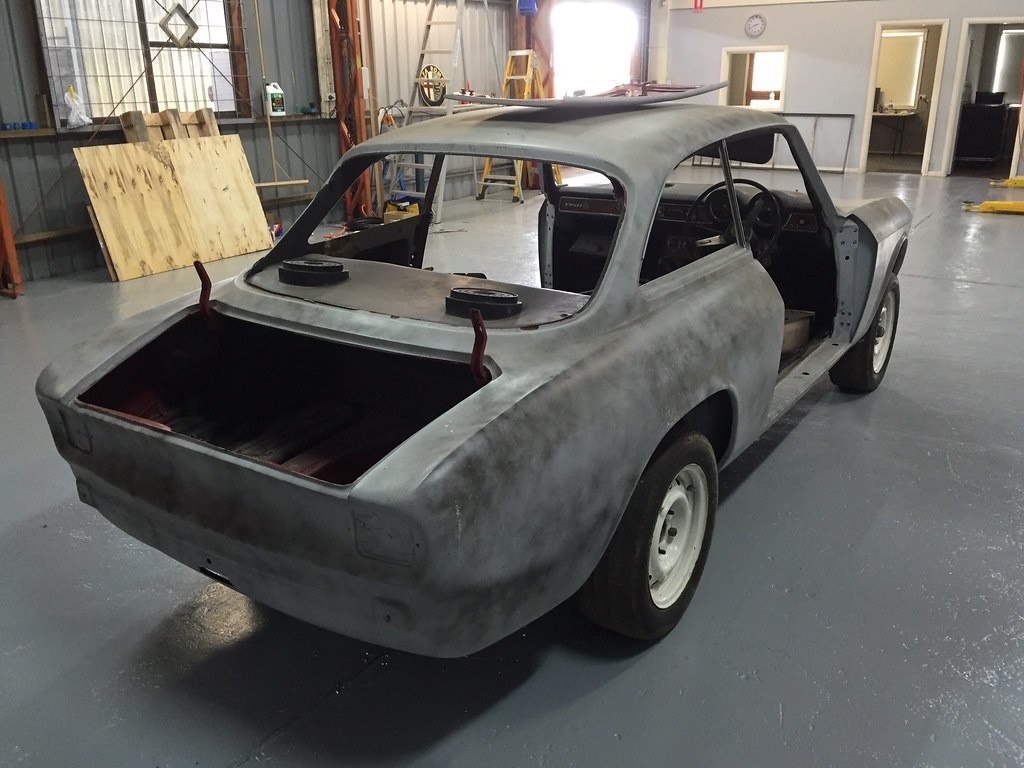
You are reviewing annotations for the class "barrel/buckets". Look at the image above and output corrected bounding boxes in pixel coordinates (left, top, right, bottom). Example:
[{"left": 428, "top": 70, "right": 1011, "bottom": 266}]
[{"left": 262, "top": 82, "right": 286, "bottom": 117}]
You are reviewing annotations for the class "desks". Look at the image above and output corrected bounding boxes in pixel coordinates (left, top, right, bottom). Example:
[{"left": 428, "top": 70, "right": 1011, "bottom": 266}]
[{"left": 873, "top": 111, "right": 916, "bottom": 158}]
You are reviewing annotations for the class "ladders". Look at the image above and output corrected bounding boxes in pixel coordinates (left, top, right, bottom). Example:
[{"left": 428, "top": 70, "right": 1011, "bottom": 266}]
[
  {"left": 387, "top": 0, "right": 503, "bottom": 224},
  {"left": 477, "top": 50, "right": 562, "bottom": 203}
]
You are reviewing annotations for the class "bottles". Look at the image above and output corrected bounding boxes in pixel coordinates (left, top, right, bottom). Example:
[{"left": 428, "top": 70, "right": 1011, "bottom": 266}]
[
  {"left": 769, "top": 92, "right": 775, "bottom": 100},
  {"left": 888, "top": 100, "right": 893, "bottom": 113}
]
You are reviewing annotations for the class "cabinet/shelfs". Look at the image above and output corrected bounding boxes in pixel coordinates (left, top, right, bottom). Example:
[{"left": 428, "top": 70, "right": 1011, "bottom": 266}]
[{"left": 955, "top": 103, "right": 1012, "bottom": 171}]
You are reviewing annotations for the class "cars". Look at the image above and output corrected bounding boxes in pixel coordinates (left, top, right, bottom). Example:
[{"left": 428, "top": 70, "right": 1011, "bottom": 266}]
[{"left": 34, "top": 101, "right": 915, "bottom": 661}]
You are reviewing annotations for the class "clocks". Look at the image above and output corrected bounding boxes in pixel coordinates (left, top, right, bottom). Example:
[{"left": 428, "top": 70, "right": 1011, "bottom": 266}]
[{"left": 745, "top": 13, "right": 767, "bottom": 36}]
